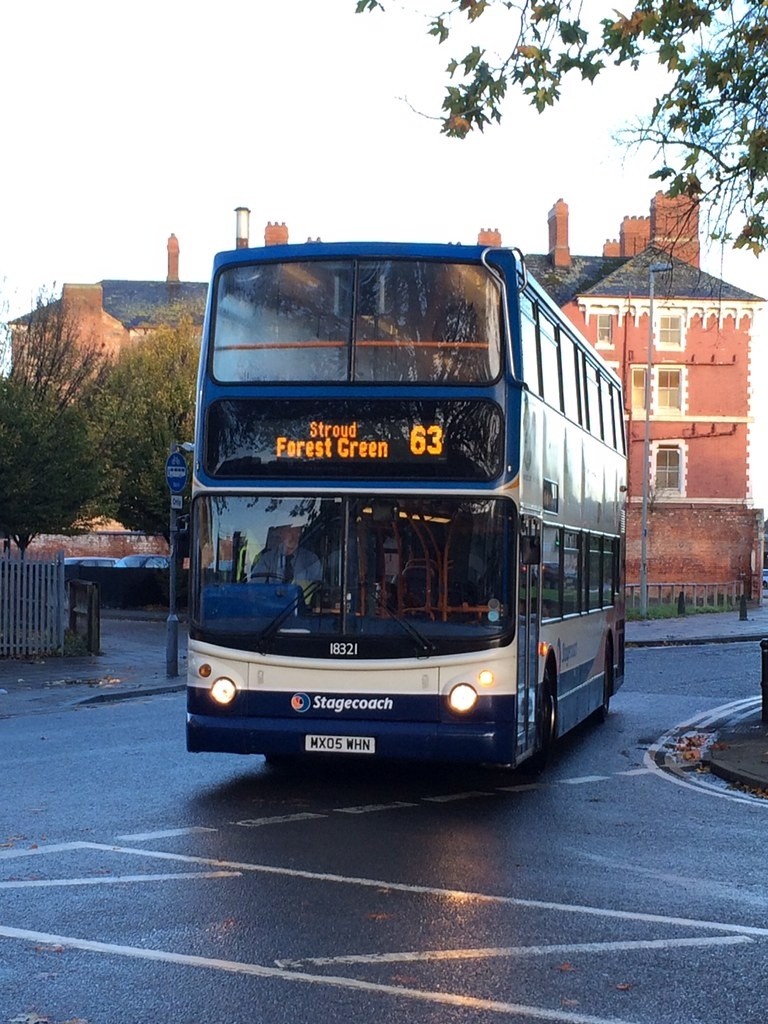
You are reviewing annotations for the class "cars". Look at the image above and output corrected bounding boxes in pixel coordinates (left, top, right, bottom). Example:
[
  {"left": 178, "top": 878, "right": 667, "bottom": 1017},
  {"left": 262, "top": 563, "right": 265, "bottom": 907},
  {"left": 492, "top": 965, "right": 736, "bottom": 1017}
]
[
  {"left": 64, "top": 557, "right": 121, "bottom": 567},
  {"left": 112, "top": 553, "right": 169, "bottom": 569},
  {"left": 208, "top": 559, "right": 233, "bottom": 573},
  {"left": 762, "top": 569, "right": 768, "bottom": 587}
]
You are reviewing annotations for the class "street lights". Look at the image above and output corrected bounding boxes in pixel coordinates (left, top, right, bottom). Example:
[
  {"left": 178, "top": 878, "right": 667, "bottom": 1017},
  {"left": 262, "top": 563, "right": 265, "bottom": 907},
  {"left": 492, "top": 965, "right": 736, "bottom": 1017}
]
[{"left": 639, "top": 261, "right": 672, "bottom": 624}]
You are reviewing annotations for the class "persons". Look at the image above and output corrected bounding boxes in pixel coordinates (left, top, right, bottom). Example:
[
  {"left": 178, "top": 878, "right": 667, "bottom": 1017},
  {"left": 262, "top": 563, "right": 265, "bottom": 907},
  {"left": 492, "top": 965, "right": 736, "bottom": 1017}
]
[{"left": 248, "top": 524, "right": 323, "bottom": 604}]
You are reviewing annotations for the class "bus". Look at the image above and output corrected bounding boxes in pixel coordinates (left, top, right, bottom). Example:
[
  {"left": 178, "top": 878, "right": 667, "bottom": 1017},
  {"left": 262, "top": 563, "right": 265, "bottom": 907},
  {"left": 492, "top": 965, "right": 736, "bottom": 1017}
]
[{"left": 181, "top": 239, "right": 631, "bottom": 784}]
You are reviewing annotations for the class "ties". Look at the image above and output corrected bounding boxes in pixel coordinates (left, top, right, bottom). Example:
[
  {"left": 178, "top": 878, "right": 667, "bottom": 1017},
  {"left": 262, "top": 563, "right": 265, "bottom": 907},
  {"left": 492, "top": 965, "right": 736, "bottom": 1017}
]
[{"left": 281, "top": 553, "right": 294, "bottom": 584}]
